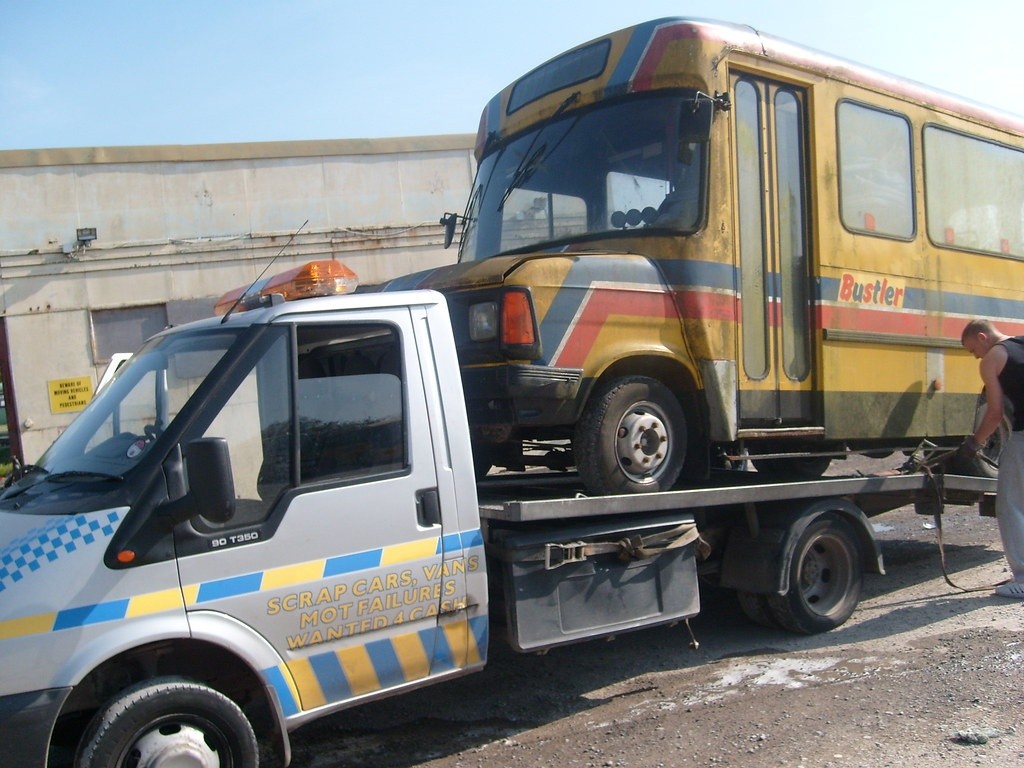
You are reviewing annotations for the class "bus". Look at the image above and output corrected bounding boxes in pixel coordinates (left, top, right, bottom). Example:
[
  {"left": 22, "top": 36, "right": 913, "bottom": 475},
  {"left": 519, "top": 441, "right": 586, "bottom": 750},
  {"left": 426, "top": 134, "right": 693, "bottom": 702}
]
[{"left": 370, "top": 16, "right": 1023, "bottom": 495}]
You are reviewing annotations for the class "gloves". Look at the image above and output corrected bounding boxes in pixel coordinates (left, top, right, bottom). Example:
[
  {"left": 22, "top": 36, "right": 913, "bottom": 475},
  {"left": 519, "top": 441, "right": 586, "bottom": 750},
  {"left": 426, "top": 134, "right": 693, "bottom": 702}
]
[{"left": 954, "top": 433, "right": 987, "bottom": 467}]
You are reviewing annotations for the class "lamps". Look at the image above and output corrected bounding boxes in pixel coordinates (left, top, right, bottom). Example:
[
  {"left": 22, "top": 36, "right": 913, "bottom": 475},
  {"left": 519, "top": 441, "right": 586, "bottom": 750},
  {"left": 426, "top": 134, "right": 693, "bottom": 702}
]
[
  {"left": 76, "top": 228, "right": 97, "bottom": 248},
  {"left": 534, "top": 197, "right": 546, "bottom": 210}
]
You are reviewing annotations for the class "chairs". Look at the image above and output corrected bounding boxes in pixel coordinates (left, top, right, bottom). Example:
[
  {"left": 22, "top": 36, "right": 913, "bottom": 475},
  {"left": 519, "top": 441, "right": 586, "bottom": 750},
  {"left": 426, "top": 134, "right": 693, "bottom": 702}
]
[{"left": 257, "top": 357, "right": 392, "bottom": 500}]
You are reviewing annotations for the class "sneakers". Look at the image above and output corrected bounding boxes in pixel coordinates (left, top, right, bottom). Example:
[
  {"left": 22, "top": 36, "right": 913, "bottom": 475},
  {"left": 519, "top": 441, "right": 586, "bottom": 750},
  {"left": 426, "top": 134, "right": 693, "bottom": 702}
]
[{"left": 995, "top": 580, "right": 1024, "bottom": 598}]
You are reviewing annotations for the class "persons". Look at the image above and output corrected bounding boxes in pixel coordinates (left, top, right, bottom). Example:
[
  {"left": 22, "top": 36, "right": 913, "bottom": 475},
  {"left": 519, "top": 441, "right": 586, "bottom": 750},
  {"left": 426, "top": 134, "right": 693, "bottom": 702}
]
[{"left": 954, "top": 319, "right": 1024, "bottom": 598}]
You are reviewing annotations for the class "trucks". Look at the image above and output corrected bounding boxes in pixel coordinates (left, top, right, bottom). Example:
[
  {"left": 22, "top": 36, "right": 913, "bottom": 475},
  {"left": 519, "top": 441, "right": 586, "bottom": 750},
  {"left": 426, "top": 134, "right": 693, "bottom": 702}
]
[{"left": 0, "top": 258, "right": 1001, "bottom": 768}]
